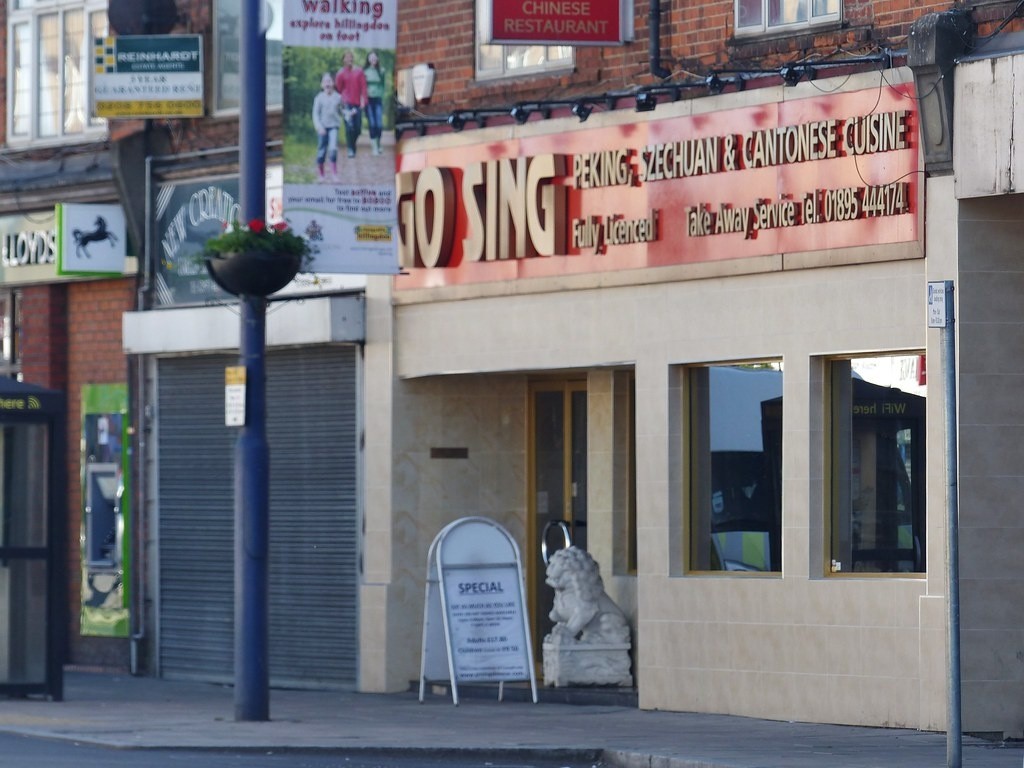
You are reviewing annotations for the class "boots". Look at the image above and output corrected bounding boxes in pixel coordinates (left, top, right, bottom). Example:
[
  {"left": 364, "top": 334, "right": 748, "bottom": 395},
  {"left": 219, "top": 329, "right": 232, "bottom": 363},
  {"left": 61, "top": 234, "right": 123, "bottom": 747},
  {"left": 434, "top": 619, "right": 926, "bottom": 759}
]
[
  {"left": 370, "top": 135, "right": 383, "bottom": 157},
  {"left": 330, "top": 161, "right": 339, "bottom": 176},
  {"left": 317, "top": 162, "right": 326, "bottom": 177}
]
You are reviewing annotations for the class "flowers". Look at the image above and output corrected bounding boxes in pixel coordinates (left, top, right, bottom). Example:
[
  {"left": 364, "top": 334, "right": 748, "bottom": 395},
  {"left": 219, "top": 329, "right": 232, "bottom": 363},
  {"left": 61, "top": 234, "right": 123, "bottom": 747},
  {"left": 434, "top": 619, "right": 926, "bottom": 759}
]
[{"left": 200, "top": 216, "right": 304, "bottom": 260}]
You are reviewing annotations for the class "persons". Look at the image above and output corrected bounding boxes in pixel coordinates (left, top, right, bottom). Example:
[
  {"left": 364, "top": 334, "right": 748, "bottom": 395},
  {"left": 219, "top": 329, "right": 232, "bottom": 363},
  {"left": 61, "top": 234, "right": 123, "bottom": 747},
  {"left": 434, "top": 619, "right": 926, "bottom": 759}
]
[
  {"left": 335, "top": 52, "right": 368, "bottom": 156},
  {"left": 364, "top": 51, "right": 385, "bottom": 155},
  {"left": 311, "top": 72, "right": 348, "bottom": 179}
]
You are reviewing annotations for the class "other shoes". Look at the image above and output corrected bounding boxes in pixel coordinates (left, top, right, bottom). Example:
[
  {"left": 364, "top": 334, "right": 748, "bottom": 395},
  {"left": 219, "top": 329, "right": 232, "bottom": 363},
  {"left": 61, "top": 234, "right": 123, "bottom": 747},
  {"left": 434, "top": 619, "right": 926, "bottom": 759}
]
[{"left": 346, "top": 143, "right": 356, "bottom": 159}]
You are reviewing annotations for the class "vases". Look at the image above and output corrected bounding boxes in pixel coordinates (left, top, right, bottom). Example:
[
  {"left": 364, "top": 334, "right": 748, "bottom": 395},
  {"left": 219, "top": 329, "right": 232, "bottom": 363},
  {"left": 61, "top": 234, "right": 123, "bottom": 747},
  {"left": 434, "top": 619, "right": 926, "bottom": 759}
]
[{"left": 207, "top": 256, "right": 300, "bottom": 297}]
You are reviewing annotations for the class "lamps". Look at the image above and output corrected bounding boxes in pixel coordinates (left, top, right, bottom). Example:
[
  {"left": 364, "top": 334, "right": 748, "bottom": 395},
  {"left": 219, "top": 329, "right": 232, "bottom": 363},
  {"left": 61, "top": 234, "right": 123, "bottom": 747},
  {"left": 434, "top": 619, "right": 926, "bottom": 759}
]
[
  {"left": 636, "top": 93, "right": 656, "bottom": 111},
  {"left": 448, "top": 113, "right": 468, "bottom": 131},
  {"left": 510, "top": 106, "right": 530, "bottom": 124},
  {"left": 705, "top": 75, "right": 725, "bottom": 94},
  {"left": 780, "top": 67, "right": 802, "bottom": 87},
  {"left": 573, "top": 103, "right": 594, "bottom": 121}
]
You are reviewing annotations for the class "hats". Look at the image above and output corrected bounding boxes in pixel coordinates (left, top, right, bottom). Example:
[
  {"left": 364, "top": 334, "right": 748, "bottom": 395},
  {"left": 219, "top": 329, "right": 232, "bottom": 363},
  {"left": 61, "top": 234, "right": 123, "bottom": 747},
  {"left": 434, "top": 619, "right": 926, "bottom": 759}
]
[{"left": 320, "top": 72, "right": 335, "bottom": 89}]
